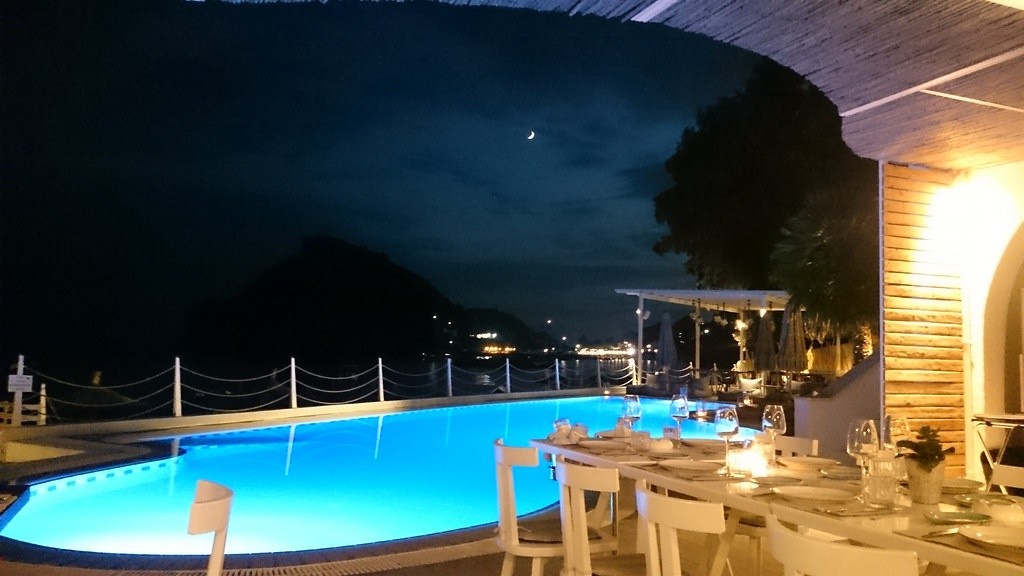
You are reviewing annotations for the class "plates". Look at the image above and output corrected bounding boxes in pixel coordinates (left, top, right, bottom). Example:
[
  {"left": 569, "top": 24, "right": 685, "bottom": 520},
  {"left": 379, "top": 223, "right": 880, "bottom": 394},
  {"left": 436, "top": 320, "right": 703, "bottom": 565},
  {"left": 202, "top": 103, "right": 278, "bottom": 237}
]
[
  {"left": 772, "top": 486, "right": 854, "bottom": 501},
  {"left": 956, "top": 495, "right": 1017, "bottom": 507},
  {"left": 821, "top": 466, "right": 861, "bottom": 480},
  {"left": 659, "top": 459, "right": 724, "bottom": 472},
  {"left": 959, "top": 525, "right": 1024, "bottom": 550},
  {"left": 575, "top": 440, "right": 628, "bottom": 449},
  {"left": 750, "top": 476, "right": 803, "bottom": 485},
  {"left": 925, "top": 512, "right": 993, "bottom": 525},
  {"left": 942, "top": 477, "right": 984, "bottom": 493}
]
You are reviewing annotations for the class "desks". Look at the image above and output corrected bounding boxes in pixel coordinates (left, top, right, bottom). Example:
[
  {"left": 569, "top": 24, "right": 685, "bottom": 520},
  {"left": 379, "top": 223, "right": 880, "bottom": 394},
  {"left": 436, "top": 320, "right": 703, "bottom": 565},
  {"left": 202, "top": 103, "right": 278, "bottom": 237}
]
[
  {"left": 970, "top": 413, "right": 1024, "bottom": 496},
  {"left": 529, "top": 436, "right": 1020, "bottom": 574}
]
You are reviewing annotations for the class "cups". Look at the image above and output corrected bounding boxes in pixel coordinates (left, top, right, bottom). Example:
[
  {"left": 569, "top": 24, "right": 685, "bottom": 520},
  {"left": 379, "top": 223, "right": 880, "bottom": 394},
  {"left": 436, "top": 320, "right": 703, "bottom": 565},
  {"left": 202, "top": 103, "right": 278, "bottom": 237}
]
[
  {"left": 865, "top": 451, "right": 898, "bottom": 511},
  {"left": 882, "top": 414, "right": 912, "bottom": 451}
]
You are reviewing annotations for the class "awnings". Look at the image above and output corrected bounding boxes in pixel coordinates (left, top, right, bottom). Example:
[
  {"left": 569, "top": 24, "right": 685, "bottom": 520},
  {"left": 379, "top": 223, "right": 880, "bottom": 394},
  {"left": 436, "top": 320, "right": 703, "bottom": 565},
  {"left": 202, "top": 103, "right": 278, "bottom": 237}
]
[{"left": 615, "top": 287, "right": 808, "bottom": 312}]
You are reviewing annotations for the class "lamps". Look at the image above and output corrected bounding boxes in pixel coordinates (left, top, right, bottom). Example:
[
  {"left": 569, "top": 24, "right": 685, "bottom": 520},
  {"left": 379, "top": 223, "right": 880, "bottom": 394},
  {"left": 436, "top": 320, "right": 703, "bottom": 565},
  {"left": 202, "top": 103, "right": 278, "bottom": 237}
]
[{"left": 638, "top": 296, "right": 778, "bottom": 334}]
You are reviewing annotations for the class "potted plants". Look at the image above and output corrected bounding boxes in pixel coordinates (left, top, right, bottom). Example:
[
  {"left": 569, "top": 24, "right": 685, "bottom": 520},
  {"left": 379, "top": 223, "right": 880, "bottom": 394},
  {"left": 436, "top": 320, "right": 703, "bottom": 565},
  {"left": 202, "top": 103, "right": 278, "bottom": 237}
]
[{"left": 893, "top": 424, "right": 955, "bottom": 519}]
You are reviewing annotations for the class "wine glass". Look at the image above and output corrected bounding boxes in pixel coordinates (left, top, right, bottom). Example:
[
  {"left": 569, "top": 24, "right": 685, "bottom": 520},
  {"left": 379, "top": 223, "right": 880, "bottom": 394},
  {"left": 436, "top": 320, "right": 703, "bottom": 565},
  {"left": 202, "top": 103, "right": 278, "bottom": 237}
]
[
  {"left": 623, "top": 394, "right": 640, "bottom": 430},
  {"left": 762, "top": 405, "right": 787, "bottom": 465},
  {"left": 670, "top": 395, "right": 688, "bottom": 441},
  {"left": 714, "top": 406, "right": 739, "bottom": 477},
  {"left": 846, "top": 419, "right": 879, "bottom": 506}
]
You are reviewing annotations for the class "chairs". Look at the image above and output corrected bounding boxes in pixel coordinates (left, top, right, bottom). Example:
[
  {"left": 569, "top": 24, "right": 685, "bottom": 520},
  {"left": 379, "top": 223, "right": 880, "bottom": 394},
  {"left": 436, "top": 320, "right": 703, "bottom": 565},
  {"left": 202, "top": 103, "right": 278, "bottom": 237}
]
[
  {"left": 494, "top": 422, "right": 1024, "bottom": 576},
  {"left": 188, "top": 479, "right": 232, "bottom": 576}
]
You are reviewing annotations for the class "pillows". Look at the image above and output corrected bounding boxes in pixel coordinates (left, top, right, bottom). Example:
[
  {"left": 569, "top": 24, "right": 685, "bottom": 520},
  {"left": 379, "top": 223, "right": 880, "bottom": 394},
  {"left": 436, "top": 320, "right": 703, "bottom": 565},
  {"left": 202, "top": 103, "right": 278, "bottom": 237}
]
[
  {"left": 717, "top": 390, "right": 739, "bottom": 401},
  {"left": 740, "top": 378, "right": 762, "bottom": 391}
]
